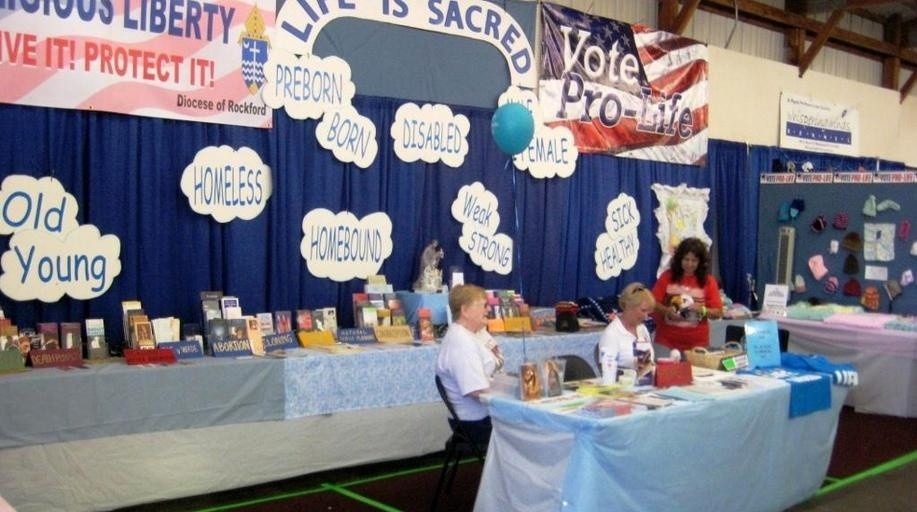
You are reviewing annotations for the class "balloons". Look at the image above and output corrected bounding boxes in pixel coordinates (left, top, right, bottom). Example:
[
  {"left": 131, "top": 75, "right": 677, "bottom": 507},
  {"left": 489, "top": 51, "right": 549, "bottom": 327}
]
[{"left": 490, "top": 102, "right": 534, "bottom": 158}]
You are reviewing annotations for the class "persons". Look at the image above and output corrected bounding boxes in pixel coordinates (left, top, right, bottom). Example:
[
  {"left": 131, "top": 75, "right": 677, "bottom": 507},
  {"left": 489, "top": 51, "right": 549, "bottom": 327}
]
[
  {"left": 651, "top": 237, "right": 723, "bottom": 361},
  {"left": 598, "top": 282, "right": 656, "bottom": 368},
  {"left": 436, "top": 284, "right": 507, "bottom": 444}
]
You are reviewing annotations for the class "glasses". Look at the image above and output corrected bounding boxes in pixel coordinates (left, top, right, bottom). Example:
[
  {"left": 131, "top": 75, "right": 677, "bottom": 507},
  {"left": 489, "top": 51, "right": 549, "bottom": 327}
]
[{"left": 468, "top": 302, "right": 487, "bottom": 308}]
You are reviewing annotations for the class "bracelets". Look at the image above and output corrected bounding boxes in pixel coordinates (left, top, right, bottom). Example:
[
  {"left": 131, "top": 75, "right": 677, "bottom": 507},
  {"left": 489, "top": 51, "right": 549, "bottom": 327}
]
[{"left": 699, "top": 305, "right": 707, "bottom": 318}]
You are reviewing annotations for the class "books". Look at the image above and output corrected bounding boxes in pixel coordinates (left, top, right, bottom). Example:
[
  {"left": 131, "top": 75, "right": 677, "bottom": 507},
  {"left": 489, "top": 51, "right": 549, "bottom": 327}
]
[
  {"left": 482, "top": 286, "right": 529, "bottom": 319},
  {"left": 314, "top": 341, "right": 366, "bottom": 355},
  {"left": 347, "top": 273, "right": 410, "bottom": 330},
  {"left": 519, "top": 359, "right": 748, "bottom": 419},
  {"left": 0, "top": 292, "right": 341, "bottom": 373}
]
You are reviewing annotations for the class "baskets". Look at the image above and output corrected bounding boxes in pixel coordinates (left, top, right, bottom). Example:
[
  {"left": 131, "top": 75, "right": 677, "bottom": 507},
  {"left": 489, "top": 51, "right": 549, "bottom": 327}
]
[{"left": 684, "top": 341, "right": 742, "bottom": 369}]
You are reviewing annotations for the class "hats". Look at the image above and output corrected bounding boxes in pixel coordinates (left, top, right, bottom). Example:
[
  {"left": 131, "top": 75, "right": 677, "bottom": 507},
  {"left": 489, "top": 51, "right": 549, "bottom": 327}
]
[
  {"left": 863, "top": 195, "right": 876, "bottom": 216},
  {"left": 833, "top": 212, "right": 848, "bottom": 229},
  {"left": 842, "top": 231, "right": 861, "bottom": 251},
  {"left": 861, "top": 286, "right": 879, "bottom": 310},
  {"left": 843, "top": 278, "right": 860, "bottom": 295},
  {"left": 843, "top": 254, "right": 858, "bottom": 273}
]
